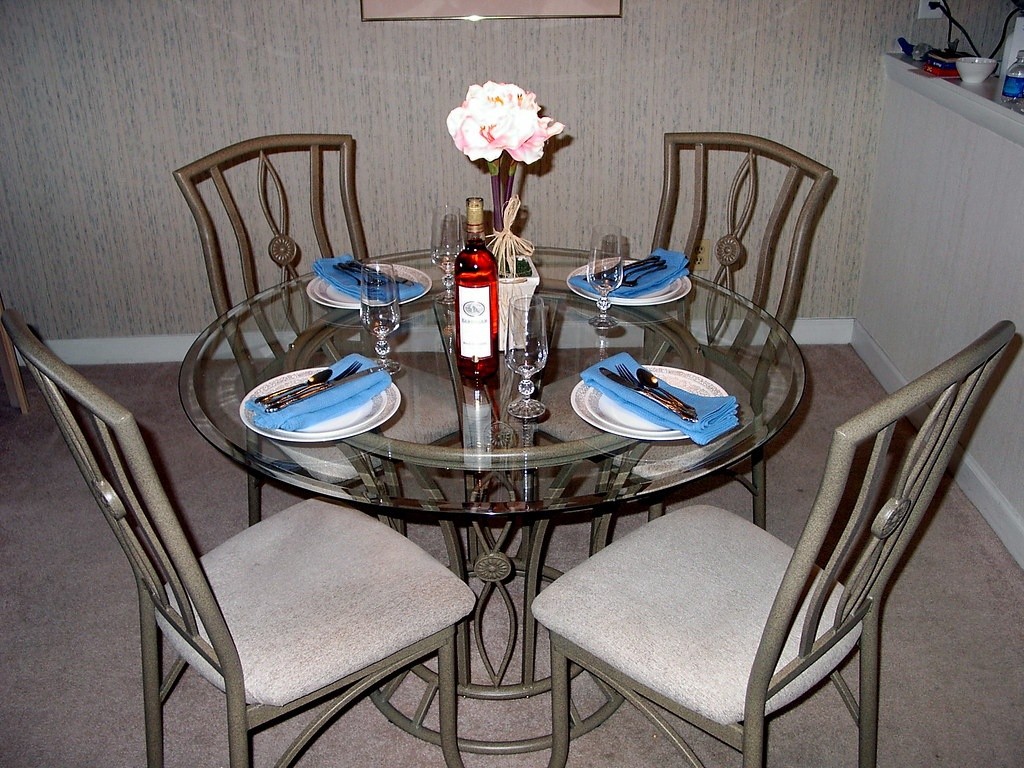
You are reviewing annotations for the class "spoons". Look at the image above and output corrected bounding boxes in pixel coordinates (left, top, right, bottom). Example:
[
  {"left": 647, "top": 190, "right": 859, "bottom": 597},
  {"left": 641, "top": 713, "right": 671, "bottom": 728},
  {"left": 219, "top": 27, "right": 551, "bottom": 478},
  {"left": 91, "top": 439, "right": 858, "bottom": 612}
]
[
  {"left": 254, "top": 369, "right": 334, "bottom": 403},
  {"left": 636, "top": 368, "right": 696, "bottom": 412},
  {"left": 333, "top": 264, "right": 373, "bottom": 288},
  {"left": 622, "top": 265, "right": 667, "bottom": 286}
]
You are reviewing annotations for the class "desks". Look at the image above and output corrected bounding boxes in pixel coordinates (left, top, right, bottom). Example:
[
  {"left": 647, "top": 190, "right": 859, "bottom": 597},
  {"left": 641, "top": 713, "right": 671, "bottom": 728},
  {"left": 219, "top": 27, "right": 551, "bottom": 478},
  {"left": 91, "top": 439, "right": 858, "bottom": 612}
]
[{"left": 178, "top": 245, "right": 806, "bottom": 755}]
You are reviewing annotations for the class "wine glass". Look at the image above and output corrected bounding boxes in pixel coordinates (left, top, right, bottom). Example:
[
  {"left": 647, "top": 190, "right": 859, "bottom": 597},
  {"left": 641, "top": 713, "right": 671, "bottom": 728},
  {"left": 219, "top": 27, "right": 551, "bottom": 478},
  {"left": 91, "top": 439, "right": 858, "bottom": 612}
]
[
  {"left": 432, "top": 205, "right": 463, "bottom": 304},
  {"left": 504, "top": 294, "right": 548, "bottom": 418},
  {"left": 359, "top": 264, "right": 401, "bottom": 375},
  {"left": 589, "top": 225, "right": 623, "bottom": 330}
]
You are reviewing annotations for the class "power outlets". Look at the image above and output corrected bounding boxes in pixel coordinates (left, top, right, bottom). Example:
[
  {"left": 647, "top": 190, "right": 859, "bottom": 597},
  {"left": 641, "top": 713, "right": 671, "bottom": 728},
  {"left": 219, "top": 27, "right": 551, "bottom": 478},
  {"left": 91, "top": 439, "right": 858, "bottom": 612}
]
[
  {"left": 690, "top": 242, "right": 710, "bottom": 270},
  {"left": 916, "top": 0, "right": 945, "bottom": 19}
]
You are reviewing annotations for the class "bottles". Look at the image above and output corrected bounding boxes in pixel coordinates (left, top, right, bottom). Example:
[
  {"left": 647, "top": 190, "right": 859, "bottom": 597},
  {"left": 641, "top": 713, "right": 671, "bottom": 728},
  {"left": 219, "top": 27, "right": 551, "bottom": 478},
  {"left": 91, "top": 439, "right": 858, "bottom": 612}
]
[
  {"left": 464, "top": 380, "right": 492, "bottom": 479},
  {"left": 455, "top": 196, "right": 499, "bottom": 380},
  {"left": 1001, "top": 50, "right": 1024, "bottom": 105}
]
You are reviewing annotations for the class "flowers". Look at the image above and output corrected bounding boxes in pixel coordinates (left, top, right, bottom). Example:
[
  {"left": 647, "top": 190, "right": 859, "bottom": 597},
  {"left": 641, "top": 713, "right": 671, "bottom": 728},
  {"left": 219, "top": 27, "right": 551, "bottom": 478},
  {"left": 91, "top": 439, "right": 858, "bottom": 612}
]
[{"left": 445, "top": 79, "right": 565, "bottom": 276}]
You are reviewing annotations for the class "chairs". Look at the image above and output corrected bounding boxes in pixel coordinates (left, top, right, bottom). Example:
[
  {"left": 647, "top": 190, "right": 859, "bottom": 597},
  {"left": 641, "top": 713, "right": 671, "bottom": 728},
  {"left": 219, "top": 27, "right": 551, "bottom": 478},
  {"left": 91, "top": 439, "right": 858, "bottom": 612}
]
[
  {"left": 171, "top": 133, "right": 489, "bottom": 536},
  {"left": 531, "top": 321, "right": 1014, "bottom": 768},
  {"left": 533, "top": 132, "right": 835, "bottom": 531},
  {"left": 0, "top": 309, "right": 478, "bottom": 768}
]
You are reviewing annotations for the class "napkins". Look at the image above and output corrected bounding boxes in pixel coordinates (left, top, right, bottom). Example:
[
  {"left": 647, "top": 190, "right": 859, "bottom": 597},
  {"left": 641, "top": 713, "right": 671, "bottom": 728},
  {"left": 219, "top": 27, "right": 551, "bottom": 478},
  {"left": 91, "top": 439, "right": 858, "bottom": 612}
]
[
  {"left": 580, "top": 352, "right": 739, "bottom": 446},
  {"left": 569, "top": 247, "right": 691, "bottom": 299},
  {"left": 245, "top": 354, "right": 392, "bottom": 432},
  {"left": 313, "top": 254, "right": 426, "bottom": 302}
]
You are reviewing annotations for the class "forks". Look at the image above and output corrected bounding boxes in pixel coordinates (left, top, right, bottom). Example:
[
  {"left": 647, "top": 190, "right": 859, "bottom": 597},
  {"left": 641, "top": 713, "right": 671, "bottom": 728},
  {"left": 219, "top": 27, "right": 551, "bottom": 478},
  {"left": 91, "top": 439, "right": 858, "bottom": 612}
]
[
  {"left": 615, "top": 363, "right": 698, "bottom": 419},
  {"left": 265, "top": 361, "right": 363, "bottom": 408}
]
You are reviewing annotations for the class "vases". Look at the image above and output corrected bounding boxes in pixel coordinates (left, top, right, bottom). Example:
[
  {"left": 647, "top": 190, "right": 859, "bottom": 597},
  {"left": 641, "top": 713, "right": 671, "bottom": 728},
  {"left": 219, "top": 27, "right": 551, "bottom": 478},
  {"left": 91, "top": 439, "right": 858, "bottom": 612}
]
[{"left": 498, "top": 256, "right": 541, "bottom": 352}]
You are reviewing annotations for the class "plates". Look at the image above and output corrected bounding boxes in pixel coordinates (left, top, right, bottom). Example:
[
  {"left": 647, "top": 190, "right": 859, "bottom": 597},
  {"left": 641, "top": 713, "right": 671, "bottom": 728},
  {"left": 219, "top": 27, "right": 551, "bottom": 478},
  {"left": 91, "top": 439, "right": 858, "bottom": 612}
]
[
  {"left": 306, "top": 264, "right": 432, "bottom": 309},
  {"left": 566, "top": 258, "right": 692, "bottom": 306},
  {"left": 239, "top": 367, "right": 401, "bottom": 443},
  {"left": 570, "top": 365, "right": 729, "bottom": 440}
]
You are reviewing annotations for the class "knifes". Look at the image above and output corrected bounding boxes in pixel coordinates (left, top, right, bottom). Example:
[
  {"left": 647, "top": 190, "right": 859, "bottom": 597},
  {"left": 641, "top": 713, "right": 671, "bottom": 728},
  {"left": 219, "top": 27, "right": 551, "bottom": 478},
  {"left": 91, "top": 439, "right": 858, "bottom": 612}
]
[
  {"left": 347, "top": 262, "right": 413, "bottom": 286},
  {"left": 265, "top": 366, "right": 388, "bottom": 411},
  {"left": 599, "top": 367, "right": 699, "bottom": 423},
  {"left": 582, "top": 255, "right": 660, "bottom": 280}
]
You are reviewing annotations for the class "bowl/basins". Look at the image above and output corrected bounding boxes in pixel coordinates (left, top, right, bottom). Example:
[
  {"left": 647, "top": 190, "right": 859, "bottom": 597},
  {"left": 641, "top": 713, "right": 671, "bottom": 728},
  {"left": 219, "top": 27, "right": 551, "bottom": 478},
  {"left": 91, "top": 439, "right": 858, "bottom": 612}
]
[{"left": 955, "top": 57, "right": 997, "bottom": 83}]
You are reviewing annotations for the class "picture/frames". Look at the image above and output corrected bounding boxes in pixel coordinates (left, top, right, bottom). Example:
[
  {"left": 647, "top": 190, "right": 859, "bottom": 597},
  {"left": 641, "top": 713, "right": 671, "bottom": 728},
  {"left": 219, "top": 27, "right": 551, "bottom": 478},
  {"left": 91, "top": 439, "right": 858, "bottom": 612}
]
[{"left": 359, "top": 0, "right": 623, "bottom": 22}]
